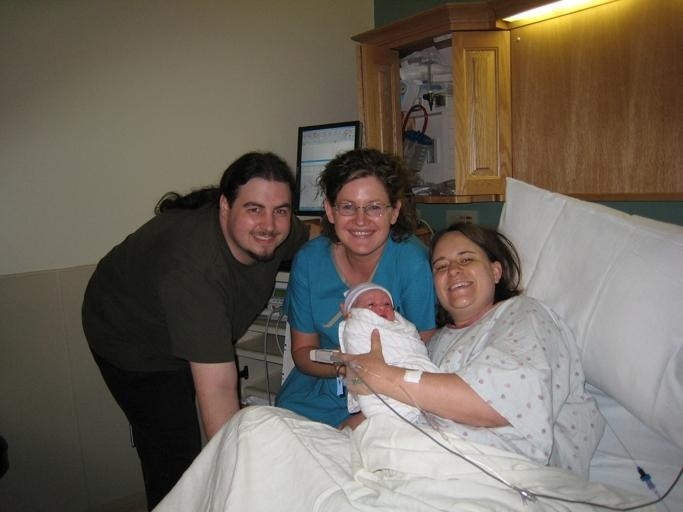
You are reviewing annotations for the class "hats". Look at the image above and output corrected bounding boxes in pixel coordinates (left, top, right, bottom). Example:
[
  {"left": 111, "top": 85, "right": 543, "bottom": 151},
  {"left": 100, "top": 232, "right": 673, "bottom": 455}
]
[{"left": 342, "top": 282, "right": 394, "bottom": 314}]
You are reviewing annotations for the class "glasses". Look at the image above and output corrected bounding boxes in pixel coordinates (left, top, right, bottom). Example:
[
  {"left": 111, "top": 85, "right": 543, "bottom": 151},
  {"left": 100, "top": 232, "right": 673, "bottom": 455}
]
[{"left": 333, "top": 199, "right": 391, "bottom": 220}]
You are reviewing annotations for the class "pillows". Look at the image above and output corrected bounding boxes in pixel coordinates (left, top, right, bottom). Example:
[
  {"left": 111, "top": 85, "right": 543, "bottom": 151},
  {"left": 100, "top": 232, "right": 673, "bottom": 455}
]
[{"left": 489, "top": 176, "right": 683, "bottom": 449}]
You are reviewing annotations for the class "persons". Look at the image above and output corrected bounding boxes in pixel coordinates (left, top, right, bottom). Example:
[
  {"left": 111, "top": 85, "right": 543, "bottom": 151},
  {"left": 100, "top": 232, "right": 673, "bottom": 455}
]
[
  {"left": 329, "top": 217, "right": 605, "bottom": 436},
  {"left": 349, "top": 282, "right": 397, "bottom": 324},
  {"left": 274, "top": 148, "right": 436, "bottom": 436},
  {"left": 81, "top": 151, "right": 320, "bottom": 512}
]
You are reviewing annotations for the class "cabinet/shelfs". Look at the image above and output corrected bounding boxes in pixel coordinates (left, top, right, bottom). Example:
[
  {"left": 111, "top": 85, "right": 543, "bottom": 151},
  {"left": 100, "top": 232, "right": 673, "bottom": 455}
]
[{"left": 346, "top": 1, "right": 511, "bottom": 203}]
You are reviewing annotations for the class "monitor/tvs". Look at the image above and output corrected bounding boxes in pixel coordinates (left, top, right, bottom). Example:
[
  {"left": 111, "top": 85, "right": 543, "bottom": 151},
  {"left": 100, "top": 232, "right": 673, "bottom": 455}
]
[{"left": 293, "top": 120, "right": 363, "bottom": 216}]
